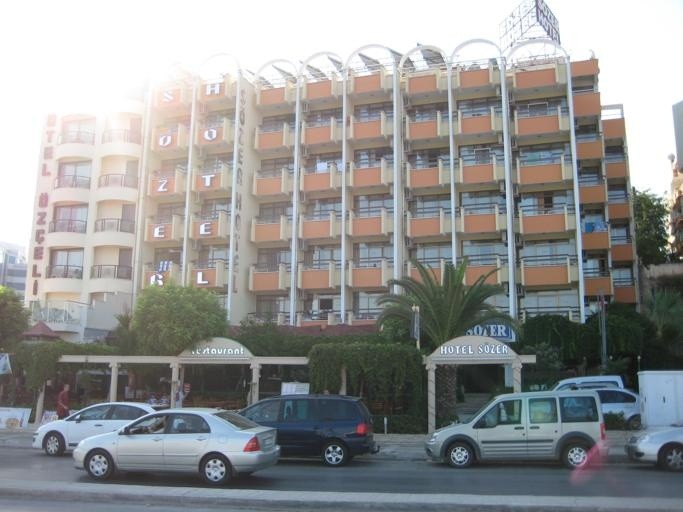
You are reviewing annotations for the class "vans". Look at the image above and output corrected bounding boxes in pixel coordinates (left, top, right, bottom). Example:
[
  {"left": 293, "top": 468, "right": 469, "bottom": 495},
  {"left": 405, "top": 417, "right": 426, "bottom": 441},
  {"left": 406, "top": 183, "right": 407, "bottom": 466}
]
[
  {"left": 549, "top": 376, "right": 624, "bottom": 391},
  {"left": 424, "top": 391, "right": 606, "bottom": 471},
  {"left": 237, "top": 393, "right": 380, "bottom": 466}
]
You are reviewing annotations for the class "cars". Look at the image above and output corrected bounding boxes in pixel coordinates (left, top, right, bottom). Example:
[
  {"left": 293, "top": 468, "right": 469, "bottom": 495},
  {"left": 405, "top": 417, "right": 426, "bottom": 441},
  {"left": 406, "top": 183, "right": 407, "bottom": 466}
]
[
  {"left": 31, "top": 401, "right": 157, "bottom": 456},
  {"left": 73, "top": 407, "right": 281, "bottom": 486},
  {"left": 624, "top": 426, "right": 683, "bottom": 471},
  {"left": 563, "top": 387, "right": 641, "bottom": 430}
]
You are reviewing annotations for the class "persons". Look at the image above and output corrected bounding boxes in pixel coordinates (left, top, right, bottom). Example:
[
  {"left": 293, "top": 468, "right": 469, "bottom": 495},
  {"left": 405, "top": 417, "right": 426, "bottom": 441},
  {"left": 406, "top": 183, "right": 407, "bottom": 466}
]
[{"left": 56, "top": 384, "right": 70, "bottom": 420}]
